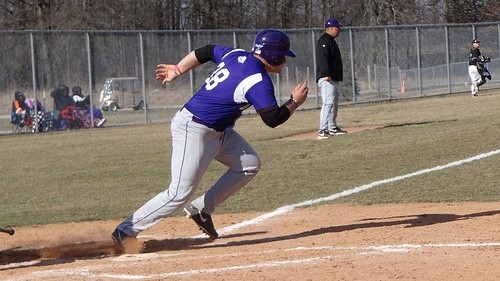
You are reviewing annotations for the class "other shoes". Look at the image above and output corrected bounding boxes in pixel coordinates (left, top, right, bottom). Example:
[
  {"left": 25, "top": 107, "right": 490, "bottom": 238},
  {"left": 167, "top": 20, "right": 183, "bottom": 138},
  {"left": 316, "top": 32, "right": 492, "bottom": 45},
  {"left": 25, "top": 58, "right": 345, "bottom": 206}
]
[
  {"left": 472, "top": 94, "right": 479, "bottom": 97},
  {"left": 471, "top": 82, "right": 476, "bottom": 92},
  {"left": 97, "top": 118, "right": 107, "bottom": 128}
]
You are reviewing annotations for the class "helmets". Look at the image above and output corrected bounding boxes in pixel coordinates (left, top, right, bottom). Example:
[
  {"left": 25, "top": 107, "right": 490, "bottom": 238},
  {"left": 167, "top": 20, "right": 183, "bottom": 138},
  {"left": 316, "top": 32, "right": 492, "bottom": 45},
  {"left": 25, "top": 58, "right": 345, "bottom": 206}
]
[{"left": 252, "top": 29, "right": 297, "bottom": 66}]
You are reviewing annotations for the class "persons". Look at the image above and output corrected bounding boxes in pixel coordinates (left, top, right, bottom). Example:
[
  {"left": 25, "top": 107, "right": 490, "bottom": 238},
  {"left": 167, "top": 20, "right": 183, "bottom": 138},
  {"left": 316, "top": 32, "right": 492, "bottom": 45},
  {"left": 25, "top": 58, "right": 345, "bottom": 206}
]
[
  {"left": 12, "top": 91, "right": 31, "bottom": 112},
  {"left": 468, "top": 38, "right": 490, "bottom": 97},
  {"left": 51, "top": 86, "right": 107, "bottom": 127},
  {"left": 112, "top": 30, "right": 309, "bottom": 252},
  {"left": 315, "top": 18, "right": 347, "bottom": 139}
]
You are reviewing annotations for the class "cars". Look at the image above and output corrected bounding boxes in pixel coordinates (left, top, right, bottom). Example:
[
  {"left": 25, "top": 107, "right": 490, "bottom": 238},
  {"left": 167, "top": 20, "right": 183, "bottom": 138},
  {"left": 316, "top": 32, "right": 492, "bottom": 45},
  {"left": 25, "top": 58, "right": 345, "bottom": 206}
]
[{"left": 100, "top": 77, "right": 148, "bottom": 112}]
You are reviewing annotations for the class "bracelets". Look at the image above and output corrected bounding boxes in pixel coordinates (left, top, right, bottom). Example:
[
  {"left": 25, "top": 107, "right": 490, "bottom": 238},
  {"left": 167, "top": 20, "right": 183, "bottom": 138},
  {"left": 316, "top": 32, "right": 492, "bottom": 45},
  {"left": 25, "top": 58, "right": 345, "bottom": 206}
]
[
  {"left": 176, "top": 65, "right": 182, "bottom": 75},
  {"left": 290, "top": 98, "right": 298, "bottom": 108}
]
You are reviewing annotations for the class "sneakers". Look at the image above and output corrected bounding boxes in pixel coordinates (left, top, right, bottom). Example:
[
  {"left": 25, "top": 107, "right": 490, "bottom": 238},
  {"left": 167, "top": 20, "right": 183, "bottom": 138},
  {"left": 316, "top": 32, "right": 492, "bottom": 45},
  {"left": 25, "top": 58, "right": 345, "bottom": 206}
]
[
  {"left": 184, "top": 201, "right": 220, "bottom": 238},
  {"left": 317, "top": 130, "right": 332, "bottom": 139},
  {"left": 111, "top": 228, "right": 137, "bottom": 252},
  {"left": 329, "top": 127, "right": 348, "bottom": 135}
]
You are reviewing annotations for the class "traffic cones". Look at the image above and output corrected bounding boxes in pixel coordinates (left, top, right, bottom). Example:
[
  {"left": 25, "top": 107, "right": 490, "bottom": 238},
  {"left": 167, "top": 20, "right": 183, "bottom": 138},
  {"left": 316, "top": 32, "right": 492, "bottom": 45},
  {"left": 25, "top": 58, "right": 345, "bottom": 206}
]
[{"left": 400, "top": 80, "right": 406, "bottom": 93}]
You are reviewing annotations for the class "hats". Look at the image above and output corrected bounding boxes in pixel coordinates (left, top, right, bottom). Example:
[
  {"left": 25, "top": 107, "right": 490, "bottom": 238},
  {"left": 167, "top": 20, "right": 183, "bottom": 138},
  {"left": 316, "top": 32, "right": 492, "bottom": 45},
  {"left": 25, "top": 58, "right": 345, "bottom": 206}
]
[
  {"left": 325, "top": 18, "right": 343, "bottom": 29},
  {"left": 474, "top": 39, "right": 480, "bottom": 43}
]
